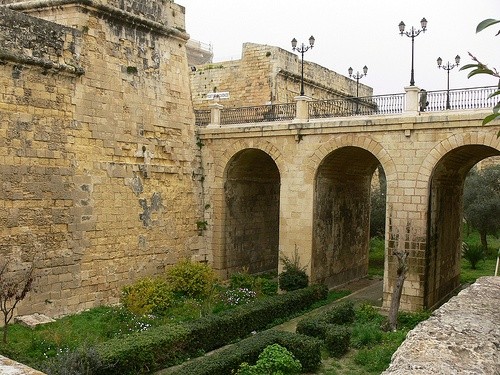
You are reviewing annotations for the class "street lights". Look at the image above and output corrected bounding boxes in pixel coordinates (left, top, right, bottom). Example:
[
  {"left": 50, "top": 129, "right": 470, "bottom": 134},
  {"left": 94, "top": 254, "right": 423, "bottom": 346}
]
[
  {"left": 349, "top": 65, "right": 368, "bottom": 114},
  {"left": 291, "top": 35, "right": 316, "bottom": 97},
  {"left": 437, "top": 54, "right": 461, "bottom": 110},
  {"left": 398, "top": 17, "right": 427, "bottom": 87}
]
